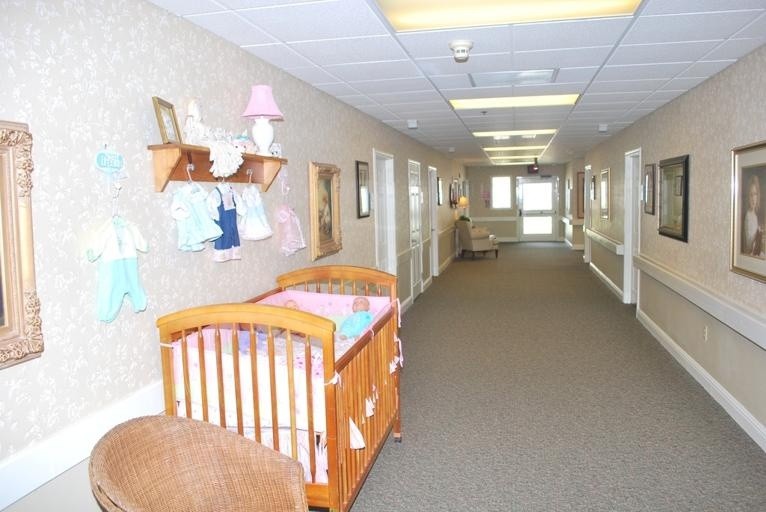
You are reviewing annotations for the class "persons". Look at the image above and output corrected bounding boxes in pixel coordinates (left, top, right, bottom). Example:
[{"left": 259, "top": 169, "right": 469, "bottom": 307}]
[
  {"left": 740, "top": 176, "right": 762, "bottom": 254},
  {"left": 319, "top": 196, "right": 333, "bottom": 232},
  {"left": 341, "top": 296, "right": 374, "bottom": 338},
  {"left": 247, "top": 298, "right": 300, "bottom": 341}
]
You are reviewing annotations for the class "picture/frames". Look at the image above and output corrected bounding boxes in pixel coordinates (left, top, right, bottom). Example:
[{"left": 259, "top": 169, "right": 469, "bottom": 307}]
[
  {"left": 437, "top": 175, "right": 470, "bottom": 209},
  {"left": 355, "top": 161, "right": 371, "bottom": 219},
  {"left": 309, "top": 160, "right": 344, "bottom": 262},
  {"left": 1, "top": 120, "right": 45, "bottom": 372},
  {"left": 637, "top": 154, "right": 691, "bottom": 244},
  {"left": 590, "top": 167, "right": 613, "bottom": 224},
  {"left": 730, "top": 138, "right": 766, "bottom": 287},
  {"left": 152, "top": 97, "right": 182, "bottom": 145}
]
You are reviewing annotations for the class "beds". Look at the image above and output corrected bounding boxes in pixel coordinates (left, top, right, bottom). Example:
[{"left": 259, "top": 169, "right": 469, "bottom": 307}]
[{"left": 155, "top": 264, "right": 403, "bottom": 512}]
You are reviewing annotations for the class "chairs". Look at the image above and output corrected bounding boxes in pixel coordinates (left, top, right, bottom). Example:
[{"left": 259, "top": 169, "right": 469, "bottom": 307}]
[
  {"left": 90, "top": 412, "right": 309, "bottom": 512},
  {"left": 455, "top": 217, "right": 500, "bottom": 259}
]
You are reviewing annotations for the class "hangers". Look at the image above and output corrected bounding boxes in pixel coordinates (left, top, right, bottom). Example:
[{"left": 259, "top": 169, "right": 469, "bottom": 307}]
[
  {"left": 109, "top": 195, "right": 121, "bottom": 218},
  {"left": 184, "top": 163, "right": 195, "bottom": 182},
  {"left": 245, "top": 167, "right": 255, "bottom": 185}
]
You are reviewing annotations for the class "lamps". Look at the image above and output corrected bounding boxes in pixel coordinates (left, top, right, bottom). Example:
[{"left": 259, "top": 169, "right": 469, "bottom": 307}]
[
  {"left": 576, "top": 172, "right": 585, "bottom": 219},
  {"left": 457, "top": 197, "right": 468, "bottom": 218},
  {"left": 240, "top": 84, "right": 286, "bottom": 158}
]
